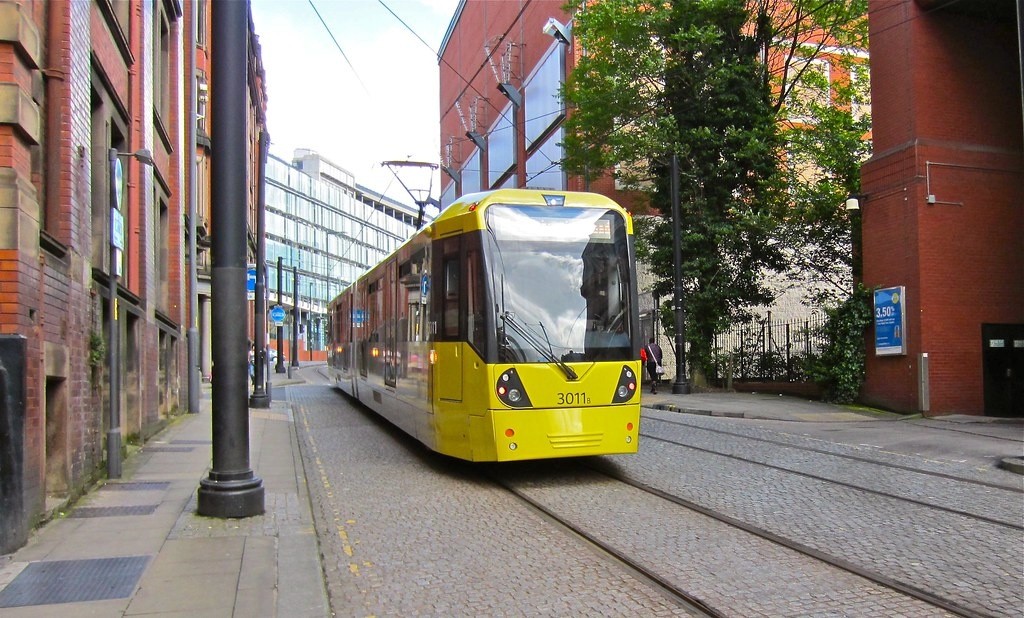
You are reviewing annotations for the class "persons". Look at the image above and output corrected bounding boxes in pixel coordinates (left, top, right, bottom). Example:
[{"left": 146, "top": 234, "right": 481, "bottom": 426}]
[
  {"left": 247, "top": 343, "right": 257, "bottom": 387},
  {"left": 645, "top": 338, "right": 664, "bottom": 395}
]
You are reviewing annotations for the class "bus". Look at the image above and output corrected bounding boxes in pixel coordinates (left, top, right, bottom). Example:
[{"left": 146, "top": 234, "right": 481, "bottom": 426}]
[{"left": 327, "top": 185, "right": 644, "bottom": 469}]
